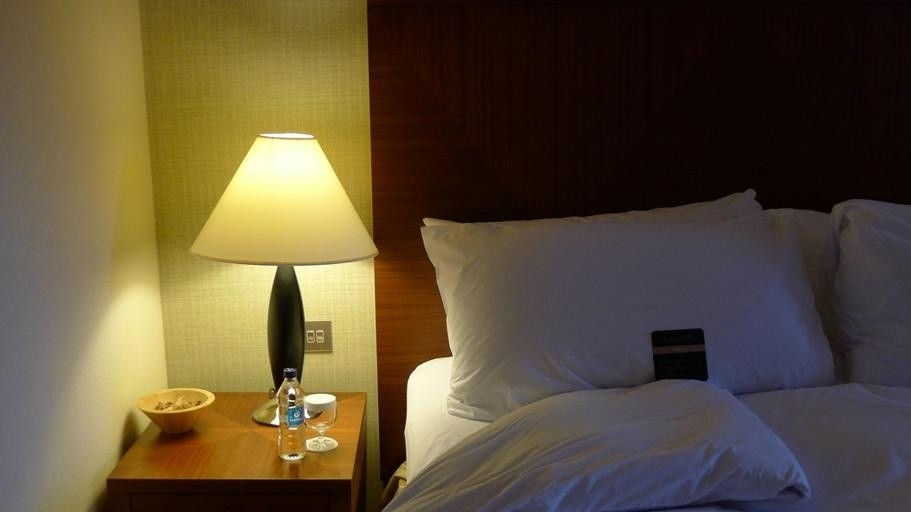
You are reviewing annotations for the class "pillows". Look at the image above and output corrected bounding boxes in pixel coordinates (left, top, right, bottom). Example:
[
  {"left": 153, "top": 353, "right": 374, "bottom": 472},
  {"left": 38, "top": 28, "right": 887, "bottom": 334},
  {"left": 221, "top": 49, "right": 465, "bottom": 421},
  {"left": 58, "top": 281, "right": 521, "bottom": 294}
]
[
  {"left": 420, "top": 189, "right": 765, "bottom": 227},
  {"left": 418, "top": 208, "right": 841, "bottom": 422},
  {"left": 829, "top": 194, "right": 910, "bottom": 394}
]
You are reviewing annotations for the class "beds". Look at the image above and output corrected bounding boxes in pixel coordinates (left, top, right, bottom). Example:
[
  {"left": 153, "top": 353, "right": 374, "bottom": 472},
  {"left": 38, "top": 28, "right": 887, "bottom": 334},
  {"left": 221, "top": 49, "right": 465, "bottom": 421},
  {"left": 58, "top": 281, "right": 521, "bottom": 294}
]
[{"left": 376, "top": 357, "right": 911, "bottom": 512}]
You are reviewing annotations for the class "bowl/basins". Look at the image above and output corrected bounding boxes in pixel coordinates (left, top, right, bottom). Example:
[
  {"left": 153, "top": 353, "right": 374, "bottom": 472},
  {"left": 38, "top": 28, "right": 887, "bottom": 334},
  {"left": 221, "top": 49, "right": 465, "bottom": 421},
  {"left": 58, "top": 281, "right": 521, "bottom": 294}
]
[{"left": 136, "top": 387, "right": 216, "bottom": 435}]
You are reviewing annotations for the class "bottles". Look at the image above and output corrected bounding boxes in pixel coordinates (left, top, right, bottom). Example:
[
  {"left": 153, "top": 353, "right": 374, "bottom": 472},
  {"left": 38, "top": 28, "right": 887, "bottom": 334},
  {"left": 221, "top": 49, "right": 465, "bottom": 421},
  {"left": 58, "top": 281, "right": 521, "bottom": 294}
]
[{"left": 276, "top": 367, "right": 307, "bottom": 462}]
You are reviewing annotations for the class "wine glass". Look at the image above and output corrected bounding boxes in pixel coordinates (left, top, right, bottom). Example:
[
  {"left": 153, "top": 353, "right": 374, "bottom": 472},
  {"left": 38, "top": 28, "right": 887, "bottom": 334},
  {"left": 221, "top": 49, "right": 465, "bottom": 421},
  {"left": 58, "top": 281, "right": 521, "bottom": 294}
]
[{"left": 304, "top": 393, "right": 339, "bottom": 452}]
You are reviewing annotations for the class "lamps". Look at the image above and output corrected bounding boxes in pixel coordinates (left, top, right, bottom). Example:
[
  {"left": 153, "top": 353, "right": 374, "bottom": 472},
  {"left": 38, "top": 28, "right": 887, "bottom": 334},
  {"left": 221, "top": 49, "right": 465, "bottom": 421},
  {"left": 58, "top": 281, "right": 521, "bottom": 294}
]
[{"left": 191, "top": 130, "right": 378, "bottom": 425}]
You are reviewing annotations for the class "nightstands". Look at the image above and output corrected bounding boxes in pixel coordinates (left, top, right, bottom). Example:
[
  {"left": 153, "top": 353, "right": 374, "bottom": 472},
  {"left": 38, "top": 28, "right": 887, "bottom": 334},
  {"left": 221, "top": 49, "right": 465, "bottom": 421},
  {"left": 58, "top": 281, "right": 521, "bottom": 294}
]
[{"left": 106, "top": 394, "right": 368, "bottom": 512}]
